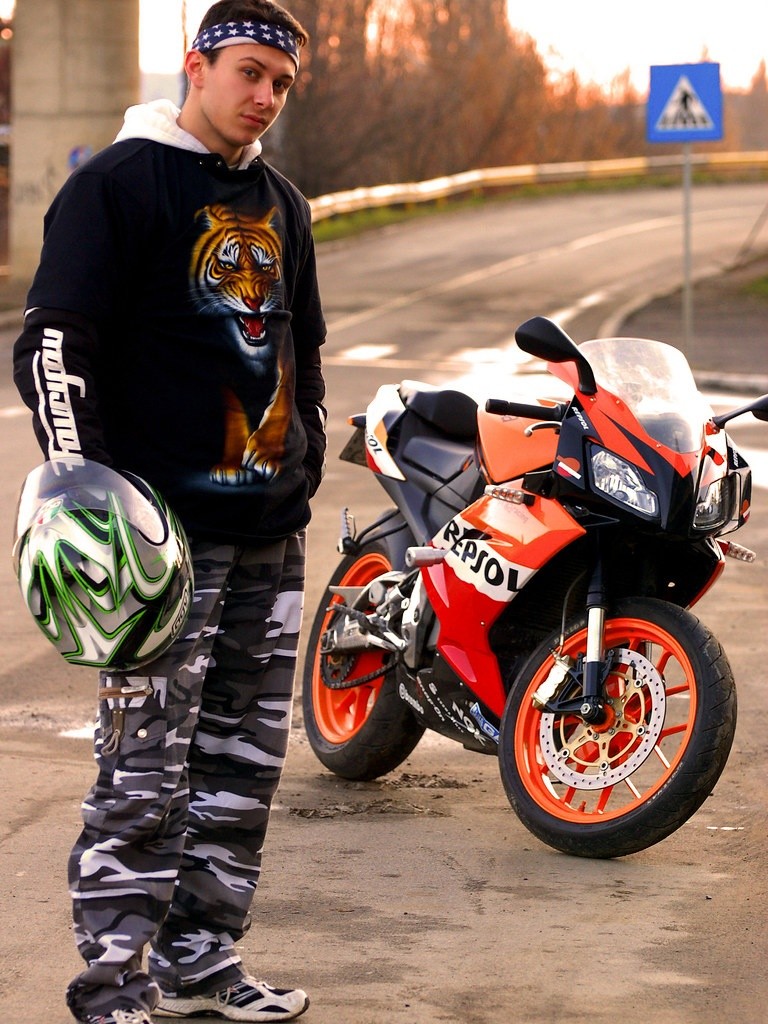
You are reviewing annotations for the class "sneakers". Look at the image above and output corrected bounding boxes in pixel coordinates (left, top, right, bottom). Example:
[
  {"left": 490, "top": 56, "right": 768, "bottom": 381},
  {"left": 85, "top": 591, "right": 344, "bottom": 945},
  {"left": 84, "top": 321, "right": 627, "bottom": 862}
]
[
  {"left": 80, "top": 1008, "right": 152, "bottom": 1023},
  {"left": 151, "top": 975, "right": 309, "bottom": 1023}
]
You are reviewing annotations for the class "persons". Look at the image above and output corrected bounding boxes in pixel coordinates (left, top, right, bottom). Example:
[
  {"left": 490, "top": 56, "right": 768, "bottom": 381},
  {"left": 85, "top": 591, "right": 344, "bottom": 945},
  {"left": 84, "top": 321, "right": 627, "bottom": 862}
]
[{"left": 11, "top": 0, "right": 328, "bottom": 1024}]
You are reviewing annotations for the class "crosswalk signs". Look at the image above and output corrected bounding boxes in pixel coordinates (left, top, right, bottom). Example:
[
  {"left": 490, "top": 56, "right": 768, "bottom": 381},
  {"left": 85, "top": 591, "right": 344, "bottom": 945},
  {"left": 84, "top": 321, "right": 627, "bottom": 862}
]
[{"left": 645, "top": 62, "right": 724, "bottom": 144}]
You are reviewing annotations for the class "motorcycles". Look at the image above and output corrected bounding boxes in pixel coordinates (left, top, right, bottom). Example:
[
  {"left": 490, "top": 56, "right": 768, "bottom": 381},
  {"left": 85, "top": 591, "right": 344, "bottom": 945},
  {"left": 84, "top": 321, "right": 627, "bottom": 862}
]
[{"left": 302, "top": 317, "right": 767, "bottom": 859}]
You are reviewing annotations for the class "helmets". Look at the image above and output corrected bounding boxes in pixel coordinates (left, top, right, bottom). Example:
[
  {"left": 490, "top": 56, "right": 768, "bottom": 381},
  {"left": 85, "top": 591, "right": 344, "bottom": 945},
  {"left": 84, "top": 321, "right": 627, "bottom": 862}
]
[{"left": 10, "top": 458, "right": 194, "bottom": 676}]
